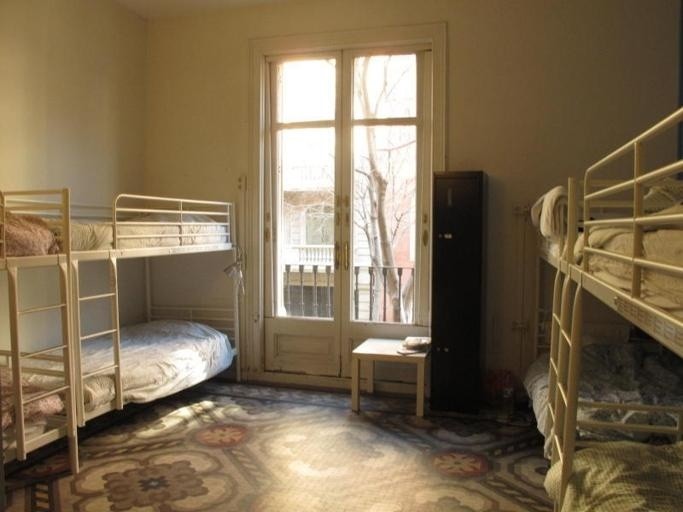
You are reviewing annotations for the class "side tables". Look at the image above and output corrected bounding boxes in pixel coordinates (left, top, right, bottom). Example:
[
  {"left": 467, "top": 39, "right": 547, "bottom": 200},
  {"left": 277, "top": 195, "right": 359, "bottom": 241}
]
[{"left": 352, "top": 338, "right": 432, "bottom": 418}]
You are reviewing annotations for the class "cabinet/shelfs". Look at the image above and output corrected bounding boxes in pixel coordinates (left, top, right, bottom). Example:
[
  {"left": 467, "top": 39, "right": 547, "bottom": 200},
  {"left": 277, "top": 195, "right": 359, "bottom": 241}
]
[{"left": 430, "top": 171, "right": 489, "bottom": 416}]
